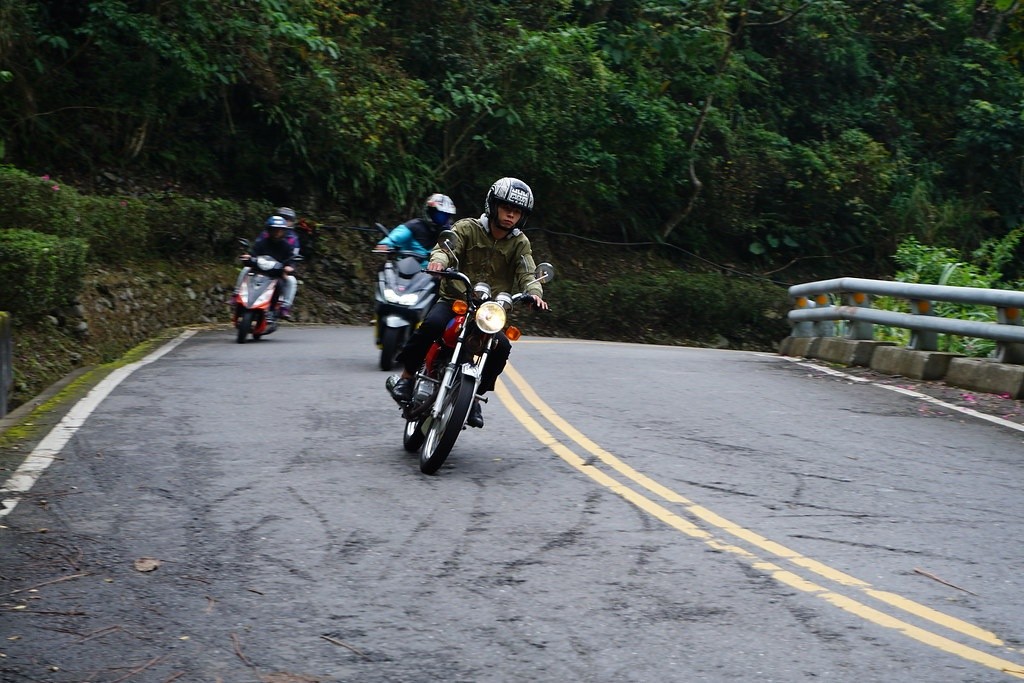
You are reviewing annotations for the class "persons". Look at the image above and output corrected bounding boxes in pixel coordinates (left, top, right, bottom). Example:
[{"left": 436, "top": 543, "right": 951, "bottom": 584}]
[
  {"left": 393, "top": 178, "right": 549, "bottom": 428},
  {"left": 225, "top": 207, "right": 302, "bottom": 320},
  {"left": 375, "top": 193, "right": 456, "bottom": 274}
]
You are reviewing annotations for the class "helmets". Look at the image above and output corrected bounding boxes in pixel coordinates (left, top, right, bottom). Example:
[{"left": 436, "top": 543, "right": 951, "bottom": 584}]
[
  {"left": 276, "top": 207, "right": 295, "bottom": 220},
  {"left": 484, "top": 176, "right": 535, "bottom": 219},
  {"left": 266, "top": 216, "right": 294, "bottom": 229},
  {"left": 422, "top": 193, "right": 456, "bottom": 227}
]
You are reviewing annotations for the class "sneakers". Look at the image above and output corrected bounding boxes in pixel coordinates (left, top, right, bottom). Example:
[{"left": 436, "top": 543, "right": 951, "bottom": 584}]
[
  {"left": 225, "top": 296, "right": 236, "bottom": 304},
  {"left": 276, "top": 307, "right": 290, "bottom": 318}
]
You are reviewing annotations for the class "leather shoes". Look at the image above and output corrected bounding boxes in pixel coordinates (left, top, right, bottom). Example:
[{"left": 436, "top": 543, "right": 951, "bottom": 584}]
[
  {"left": 391, "top": 376, "right": 412, "bottom": 400},
  {"left": 467, "top": 400, "right": 484, "bottom": 429}
]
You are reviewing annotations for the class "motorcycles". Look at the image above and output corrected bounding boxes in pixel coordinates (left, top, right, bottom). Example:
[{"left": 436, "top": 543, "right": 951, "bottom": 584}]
[
  {"left": 230, "top": 234, "right": 306, "bottom": 343},
  {"left": 385, "top": 228, "right": 557, "bottom": 476},
  {"left": 365, "top": 221, "right": 444, "bottom": 370}
]
